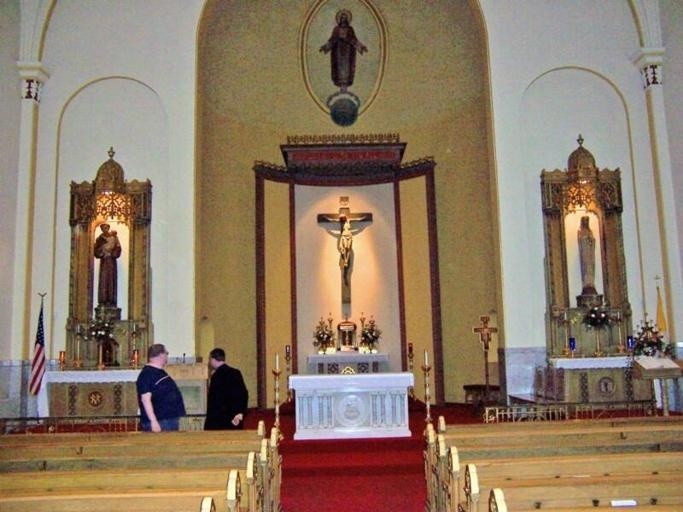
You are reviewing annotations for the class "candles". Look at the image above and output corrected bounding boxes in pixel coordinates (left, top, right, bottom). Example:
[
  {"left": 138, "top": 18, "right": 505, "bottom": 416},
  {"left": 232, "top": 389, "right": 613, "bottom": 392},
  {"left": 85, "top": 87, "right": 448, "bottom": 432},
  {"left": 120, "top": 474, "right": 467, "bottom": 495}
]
[
  {"left": 76, "top": 324, "right": 80, "bottom": 333},
  {"left": 275, "top": 353, "right": 278, "bottom": 371},
  {"left": 617, "top": 312, "right": 620, "bottom": 319},
  {"left": 564, "top": 311, "right": 567, "bottom": 320},
  {"left": 133, "top": 323, "right": 136, "bottom": 332},
  {"left": 425, "top": 350, "right": 428, "bottom": 366}
]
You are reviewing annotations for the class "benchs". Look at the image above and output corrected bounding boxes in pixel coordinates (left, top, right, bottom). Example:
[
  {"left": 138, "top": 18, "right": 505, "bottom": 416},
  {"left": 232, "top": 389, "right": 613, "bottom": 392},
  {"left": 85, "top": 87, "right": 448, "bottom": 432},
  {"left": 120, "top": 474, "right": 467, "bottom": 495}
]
[
  {"left": 423, "top": 413, "right": 683, "bottom": 512},
  {"left": 0, "top": 421, "right": 283, "bottom": 512}
]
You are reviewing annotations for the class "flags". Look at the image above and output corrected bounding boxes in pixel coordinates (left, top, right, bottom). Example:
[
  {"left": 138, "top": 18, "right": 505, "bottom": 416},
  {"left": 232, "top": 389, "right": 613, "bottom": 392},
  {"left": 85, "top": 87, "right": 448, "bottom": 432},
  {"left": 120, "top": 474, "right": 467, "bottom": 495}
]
[
  {"left": 28, "top": 307, "right": 46, "bottom": 395},
  {"left": 655, "top": 286, "right": 667, "bottom": 332}
]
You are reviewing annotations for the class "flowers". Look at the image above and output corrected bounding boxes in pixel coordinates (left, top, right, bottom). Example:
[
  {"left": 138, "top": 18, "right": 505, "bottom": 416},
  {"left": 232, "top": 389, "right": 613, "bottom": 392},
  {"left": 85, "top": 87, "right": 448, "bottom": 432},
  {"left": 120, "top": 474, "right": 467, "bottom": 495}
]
[
  {"left": 313, "top": 326, "right": 334, "bottom": 354},
  {"left": 360, "top": 325, "right": 382, "bottom": 353},
  {"left": 81, "top": 318, "right": 120, "bottom": 345},
  {"left": 632, "top": 321, "right": 673, "bottom": 357},
  {"left": 582, "top": 305, "right": 620, "bottom": 331}
]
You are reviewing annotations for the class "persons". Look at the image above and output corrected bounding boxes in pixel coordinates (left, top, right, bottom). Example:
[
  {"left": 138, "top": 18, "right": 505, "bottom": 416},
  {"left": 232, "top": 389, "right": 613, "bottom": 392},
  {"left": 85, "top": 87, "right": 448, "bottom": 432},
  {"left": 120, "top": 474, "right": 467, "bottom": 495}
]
[
  {"left": 203, "top": 348, "right": 248, "bottom": 430},
  {"left": 94, "top": 224, "right": 121, "bottom": 307},
  {"left": 322, "top": 214, "right": 367, "bottom": 279},
  {"left": 576, "top": 216, "right": 598, "bottom": 294},
  {"left": 135, "top": 343, "right": 186, "bottom": 434},
  {"left": 98, "top": 230, "right": 117, "bottom": 258},
  {"left": 319, "top": 9, "right": 368, "bottom": 91}
]
